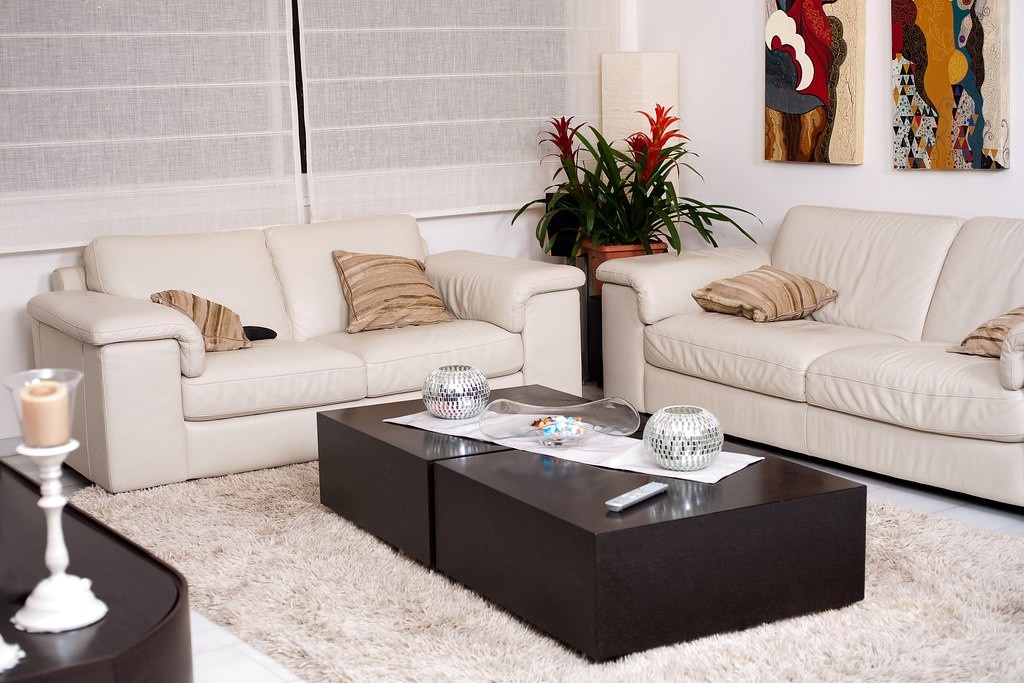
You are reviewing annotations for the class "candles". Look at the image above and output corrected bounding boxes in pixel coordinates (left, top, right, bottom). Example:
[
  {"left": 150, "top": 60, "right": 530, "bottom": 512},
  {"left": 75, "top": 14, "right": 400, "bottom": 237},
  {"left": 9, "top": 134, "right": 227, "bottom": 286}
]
[{"left": 17, "top": 380, "right": 72, "bottom": 447}]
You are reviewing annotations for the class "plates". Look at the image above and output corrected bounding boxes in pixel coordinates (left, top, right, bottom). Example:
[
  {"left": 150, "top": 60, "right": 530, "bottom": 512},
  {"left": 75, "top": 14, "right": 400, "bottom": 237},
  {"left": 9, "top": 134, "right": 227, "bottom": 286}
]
[{"left": 479, "top": 397, "right": 641, "bottom": 448}]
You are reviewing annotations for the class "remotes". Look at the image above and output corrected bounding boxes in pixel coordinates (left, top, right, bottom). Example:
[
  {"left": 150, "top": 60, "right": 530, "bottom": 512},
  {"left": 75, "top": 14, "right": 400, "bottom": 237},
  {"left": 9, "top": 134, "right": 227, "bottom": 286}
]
[{"left": 604, "top": 481, "right": 669, "bottom": 512}]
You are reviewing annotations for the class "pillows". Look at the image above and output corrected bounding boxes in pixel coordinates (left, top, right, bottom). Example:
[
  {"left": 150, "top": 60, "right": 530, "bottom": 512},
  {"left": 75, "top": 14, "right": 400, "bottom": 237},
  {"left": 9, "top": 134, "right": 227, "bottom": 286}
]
[
  {"left": 264, "top": 214, "right": 429, "bottom": 342},
  {"left": 82, "top": 231, "right": 294, "bottom": 341},
  {"left": 945, "top": 304, "right": 1024, "bottom": 358},
  {"left": 332, "top": 249, "right": 453, "bottom": 336},
  {"left": 149, "top": 290, "right": 253, "bottom": 353},
  {"left": 918, "top": 218, "right": 1024, "bottom": 345},
  {"left": 772, "top": 204, "right": 966, "bottom": 344},
  {"left": 690, "top": 265, "right": 838, "bottom": 323}
]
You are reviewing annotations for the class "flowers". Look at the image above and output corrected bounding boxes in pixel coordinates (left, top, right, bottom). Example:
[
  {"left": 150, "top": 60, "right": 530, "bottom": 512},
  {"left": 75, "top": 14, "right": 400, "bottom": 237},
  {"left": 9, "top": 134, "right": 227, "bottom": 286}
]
[{"left": 508, "top": 101, "right": 764, "bottom": 267}]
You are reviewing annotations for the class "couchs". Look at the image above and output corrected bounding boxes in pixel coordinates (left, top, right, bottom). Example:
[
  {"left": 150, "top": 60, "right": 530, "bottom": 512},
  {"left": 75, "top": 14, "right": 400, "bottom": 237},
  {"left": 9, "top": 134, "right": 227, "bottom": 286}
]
[
  {"left": 27, "top": 238, "right": 586, "bottom": 494},
  {"left": 593, "top": 245, "right": 1024, "bottom": 507}
]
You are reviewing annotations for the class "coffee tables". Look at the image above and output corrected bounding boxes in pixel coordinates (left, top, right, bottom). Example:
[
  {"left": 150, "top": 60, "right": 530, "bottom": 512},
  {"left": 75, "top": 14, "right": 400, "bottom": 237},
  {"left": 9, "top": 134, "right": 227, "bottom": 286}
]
[
  {"left": 315, "top": 384, "right": 652, "bottom": 571},
  {"left": 433, "top": 424, "right": 868, "bottom": 665}
]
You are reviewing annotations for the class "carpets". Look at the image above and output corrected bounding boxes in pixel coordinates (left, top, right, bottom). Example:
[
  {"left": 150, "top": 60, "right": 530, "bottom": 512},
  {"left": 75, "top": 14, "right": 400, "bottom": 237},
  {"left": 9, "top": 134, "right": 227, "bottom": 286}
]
[{"left": 70, "top": 458, "right": 1024, "bottom": 683}]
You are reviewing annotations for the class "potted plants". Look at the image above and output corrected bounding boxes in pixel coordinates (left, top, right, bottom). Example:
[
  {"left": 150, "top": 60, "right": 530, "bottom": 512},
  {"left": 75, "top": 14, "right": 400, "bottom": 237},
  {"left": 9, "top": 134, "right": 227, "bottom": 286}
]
[{"left": 581, "top": 235, "right": 669, "bottom": 296}]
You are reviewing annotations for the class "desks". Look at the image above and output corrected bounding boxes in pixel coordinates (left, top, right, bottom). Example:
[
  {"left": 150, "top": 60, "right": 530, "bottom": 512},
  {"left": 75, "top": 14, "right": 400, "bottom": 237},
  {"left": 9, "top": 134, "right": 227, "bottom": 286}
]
[{"left": 0, "top": 459, "right": 194, "bottom": 683}]
